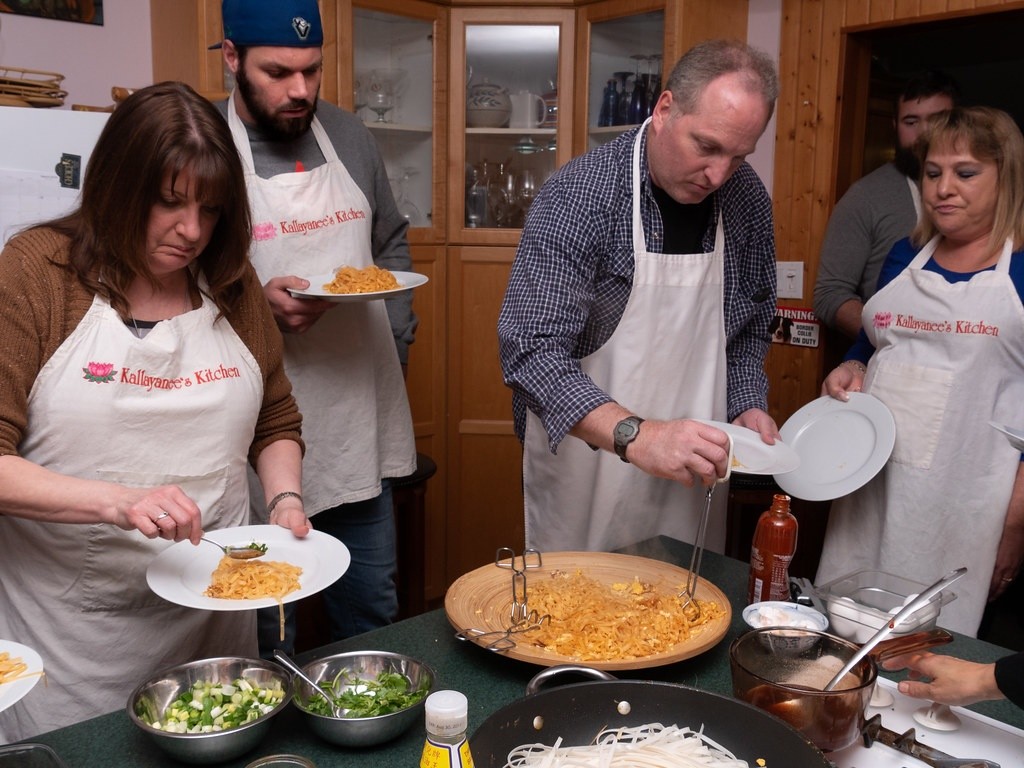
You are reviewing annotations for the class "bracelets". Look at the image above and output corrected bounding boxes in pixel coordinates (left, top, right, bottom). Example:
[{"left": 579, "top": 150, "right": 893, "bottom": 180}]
[
  {"left": 267, "top": 492, "right": 304, "bottom": 519},
  {"left": 835, "top": 359, "right": 866, "bottom": 376}
]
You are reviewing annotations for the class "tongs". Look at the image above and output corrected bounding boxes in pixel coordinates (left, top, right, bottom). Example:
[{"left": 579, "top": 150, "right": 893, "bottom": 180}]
[
  {"left": 674, "top": 427, "right": 733, "bottom": 624},
  {"left": 455, "top": 609, "right": 552, "bottom": 652},
  {"left": 495, "top": 547, "right": 542, "bottom": 624}
]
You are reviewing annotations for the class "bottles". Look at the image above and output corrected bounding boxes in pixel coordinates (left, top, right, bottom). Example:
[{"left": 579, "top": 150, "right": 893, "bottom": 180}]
[
  {"left": 418, "top": 691, "right": 474, "bottom": 768},
  {"left": 604, "top": 78, "right": 617, "bottom": 126},
  {"left": 539, "top": 78, "right": 558, "bottom": 128},
  {"left": 748, "top": 494, "right": 799, "bottom": 603}
]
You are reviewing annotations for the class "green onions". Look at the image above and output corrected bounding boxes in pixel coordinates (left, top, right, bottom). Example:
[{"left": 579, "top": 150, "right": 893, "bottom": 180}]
[{"left": 147, "top": 679, "right": 284, "bottom": 733}]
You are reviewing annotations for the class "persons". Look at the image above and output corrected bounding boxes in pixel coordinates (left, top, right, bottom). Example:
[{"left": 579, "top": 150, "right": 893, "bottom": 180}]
[
  {"left": 210, "top": 0, "right": 421, "bottom": 646},
  {"left": 822, "top": 106, "right": 1024, "bottom": 637},
  {"left": 1, "top": 81, "right": 315, "bottom": 753},
  {"left": 812, "top": 75, "right": 959, "bottom": 338},
  {"left": 498, "top": 40, "right": 778, "bottom": 561},
  {"left": 883, "top": 650, "right": 1024, "bottom": 714}
]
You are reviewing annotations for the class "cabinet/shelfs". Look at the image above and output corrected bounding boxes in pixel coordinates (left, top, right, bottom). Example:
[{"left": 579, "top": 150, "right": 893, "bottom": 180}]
[
  {"left": 573, "top": 0, "right": 751, "bottom": 161},
  {"left": 453, "top": 0, "right": 575, "bottom": 582},
  {"left": 156, "top": 1, "right": 448, "bottom": 603}
]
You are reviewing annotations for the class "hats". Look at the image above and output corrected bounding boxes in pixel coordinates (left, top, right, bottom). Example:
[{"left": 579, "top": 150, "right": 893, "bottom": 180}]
[{"left": 206, "top": 0, "right": 323, "bottom": 50}]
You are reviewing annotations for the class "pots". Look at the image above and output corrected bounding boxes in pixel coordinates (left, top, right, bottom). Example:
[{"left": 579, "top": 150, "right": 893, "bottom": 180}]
[{"left": 470, "top": 666, "right": 834, "bottom": 768}]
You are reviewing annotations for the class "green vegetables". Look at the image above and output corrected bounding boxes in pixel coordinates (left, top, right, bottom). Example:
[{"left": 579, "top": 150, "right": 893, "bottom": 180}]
[
  {"left": 248, "top": 542, "right": 269, "bottom": 552},
  {"left": 300, "top": 665, "right": 428, "bottom": 720}
]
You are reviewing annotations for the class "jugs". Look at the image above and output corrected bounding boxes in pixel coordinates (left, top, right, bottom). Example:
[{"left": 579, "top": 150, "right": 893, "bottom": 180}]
[{"left": 462, "top": 83, "right": 512, "bottom": 128}]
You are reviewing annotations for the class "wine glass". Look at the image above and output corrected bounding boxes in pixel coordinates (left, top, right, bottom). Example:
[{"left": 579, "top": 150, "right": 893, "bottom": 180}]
[
  {"left": 463, "top": 159, "right": 558, "bottom": 229},
  {"left": 615, "top": 53, "right": 665, "bottom": 124},
  {"left": 349, "top": 67, "right": 423, "bottom": 225}
]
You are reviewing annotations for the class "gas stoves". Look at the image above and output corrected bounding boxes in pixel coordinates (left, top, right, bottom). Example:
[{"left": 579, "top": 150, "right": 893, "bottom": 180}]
[{"left": 778, "top": 665, "right": 1024, "bottom": 768}]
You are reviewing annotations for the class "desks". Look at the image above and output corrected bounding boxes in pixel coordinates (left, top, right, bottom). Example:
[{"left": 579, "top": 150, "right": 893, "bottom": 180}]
[{"left": 0, "top": 535, "right": 1023, "bottom": 768}]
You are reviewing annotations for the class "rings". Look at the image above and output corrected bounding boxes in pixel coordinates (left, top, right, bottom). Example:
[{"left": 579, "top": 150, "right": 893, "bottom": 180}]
[{"left": 153, "top": 512, "right": 168, "bottom": 524}]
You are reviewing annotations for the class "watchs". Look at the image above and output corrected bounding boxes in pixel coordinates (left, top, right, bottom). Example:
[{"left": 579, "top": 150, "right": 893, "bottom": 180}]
[{"left": 614, "top": 415, "right": 645, "bottom": 466}]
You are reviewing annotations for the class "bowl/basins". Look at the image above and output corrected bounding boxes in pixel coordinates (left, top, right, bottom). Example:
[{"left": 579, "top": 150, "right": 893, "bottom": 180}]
[
  {"left": 731, "top": 625, "right": 955, "bottom": 756},
  {"left": 293, "top": 649, "right": 435, "bottom": 747},
  {"left": 128, "top": 658, "right": 292, "bottom": 759},
  {"left": 743, "top": 600, "right": 829, "bottom": 657},
  {"left": 818, "top": 566, "right": 959, "bottom": 644}
]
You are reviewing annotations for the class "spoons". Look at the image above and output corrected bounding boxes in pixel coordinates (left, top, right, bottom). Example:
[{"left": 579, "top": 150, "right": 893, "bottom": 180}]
[
  {"left": 203, "top": 537, "right": 264, "bottom": 559},
  {"left": 275, "top": 648, "right": 354, "bottom": 718}
]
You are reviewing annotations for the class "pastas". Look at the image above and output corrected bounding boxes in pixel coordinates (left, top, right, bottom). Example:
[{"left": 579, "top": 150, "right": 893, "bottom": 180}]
[
  {"left": 500, "top": 721, "right": 753, "bottom": 768},
  {"left": 209, "top": 556, "right": 302, "bottom": 640},
  {"left": 0, "top": 650, "right": 50, "bottom": 688},
  {"left": 517, "top": 569, "right": 727, "bottom": 661},
  {"left": 323, "top": 265, "right": 403, "bottom": 295}
]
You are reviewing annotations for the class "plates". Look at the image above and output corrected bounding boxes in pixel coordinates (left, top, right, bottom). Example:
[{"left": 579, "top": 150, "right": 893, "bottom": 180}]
[
  {"left": 146, "top": 523, "right": 351, "bottom": 610},
  {"left": 987, "top": 419, "right": 1023, "bottom": 451},
  {"left": 1, "top": 638, "right": 44, "bottom": 713},
  {"left": 690, "top": 418, "right": 802, "bottom": 474},
  {"left": 446, "top": 551, "right": 732, "bottom": 670},
  {"left": 775, "top": 392, "right": 896, "bottom": 502},
  {"left": 285, "top": 269, "right": 430, "bottom": 301}
]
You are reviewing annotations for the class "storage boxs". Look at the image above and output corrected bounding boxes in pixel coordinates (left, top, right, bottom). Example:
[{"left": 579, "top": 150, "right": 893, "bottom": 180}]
[{"left": 814, "top": 563, "right": 959, "bottom": 647}]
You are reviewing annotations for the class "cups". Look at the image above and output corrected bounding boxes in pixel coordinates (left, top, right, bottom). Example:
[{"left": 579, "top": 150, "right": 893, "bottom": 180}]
[{"left": 507, "top": 93, "right": 547, "bottom": 127}]
